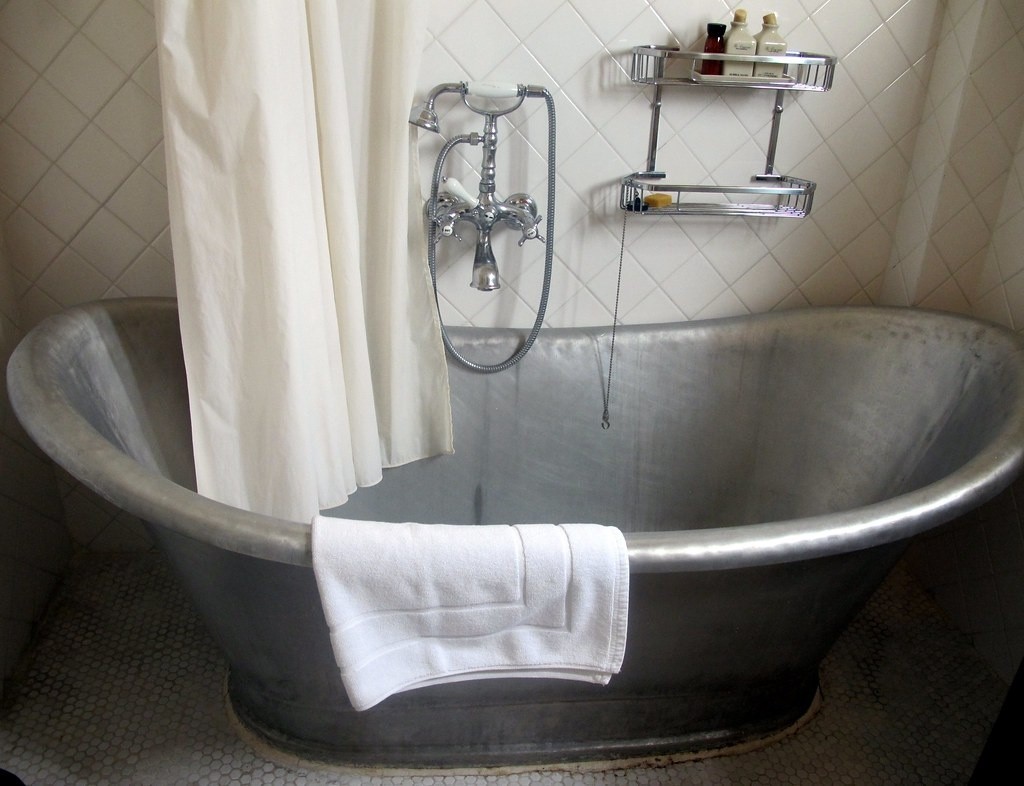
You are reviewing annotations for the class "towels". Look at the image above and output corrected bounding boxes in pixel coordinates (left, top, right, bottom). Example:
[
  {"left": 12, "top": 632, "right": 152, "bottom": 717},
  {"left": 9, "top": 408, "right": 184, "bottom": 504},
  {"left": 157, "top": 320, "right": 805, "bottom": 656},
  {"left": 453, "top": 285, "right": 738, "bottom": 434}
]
[{"left": 309, "top": 511, "right": 634, "bottom": 715}]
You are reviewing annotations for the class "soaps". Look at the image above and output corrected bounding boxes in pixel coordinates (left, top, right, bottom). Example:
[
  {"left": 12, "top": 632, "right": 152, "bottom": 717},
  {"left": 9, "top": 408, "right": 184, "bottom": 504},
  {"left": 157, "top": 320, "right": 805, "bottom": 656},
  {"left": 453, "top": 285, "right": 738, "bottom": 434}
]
[{"left": 644, "top": 192, "right": 672, "bottom": 209}]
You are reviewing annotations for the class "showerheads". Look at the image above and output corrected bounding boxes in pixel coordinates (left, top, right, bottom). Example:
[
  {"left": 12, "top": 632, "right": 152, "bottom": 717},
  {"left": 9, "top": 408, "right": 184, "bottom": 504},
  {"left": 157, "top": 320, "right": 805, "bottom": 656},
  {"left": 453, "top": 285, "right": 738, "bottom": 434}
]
[{"left": 406, "top": 77, "right": 546, "bottom": 134}]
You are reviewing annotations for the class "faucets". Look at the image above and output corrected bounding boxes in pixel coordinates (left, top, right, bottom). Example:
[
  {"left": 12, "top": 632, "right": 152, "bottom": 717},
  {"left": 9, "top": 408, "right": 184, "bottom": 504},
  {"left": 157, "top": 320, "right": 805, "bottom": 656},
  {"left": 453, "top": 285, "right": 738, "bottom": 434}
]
[{"left": 468, "top": 236, "right": 504, "bottom": 293}]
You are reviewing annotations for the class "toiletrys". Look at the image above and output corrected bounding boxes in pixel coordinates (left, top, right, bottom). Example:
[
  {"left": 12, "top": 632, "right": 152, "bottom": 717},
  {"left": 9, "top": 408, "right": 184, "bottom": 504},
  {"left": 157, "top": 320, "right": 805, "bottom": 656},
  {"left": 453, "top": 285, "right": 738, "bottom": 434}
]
[
  {"left": 701, "top": 21, "right": 728, "bottom": 78},
  {"left": 719, "top": 8, "right": 757, "bottom": 80},
  {"left": 752, "top": 12, "right": 788, "bottom": 83}
]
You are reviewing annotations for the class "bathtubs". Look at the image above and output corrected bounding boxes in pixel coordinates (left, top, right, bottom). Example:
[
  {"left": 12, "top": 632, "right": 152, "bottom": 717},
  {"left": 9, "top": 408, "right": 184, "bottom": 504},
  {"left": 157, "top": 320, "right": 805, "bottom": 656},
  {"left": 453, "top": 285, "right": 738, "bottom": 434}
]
[{"left": 3, "top": 294, "right": 1024, "bottom": 771}]
